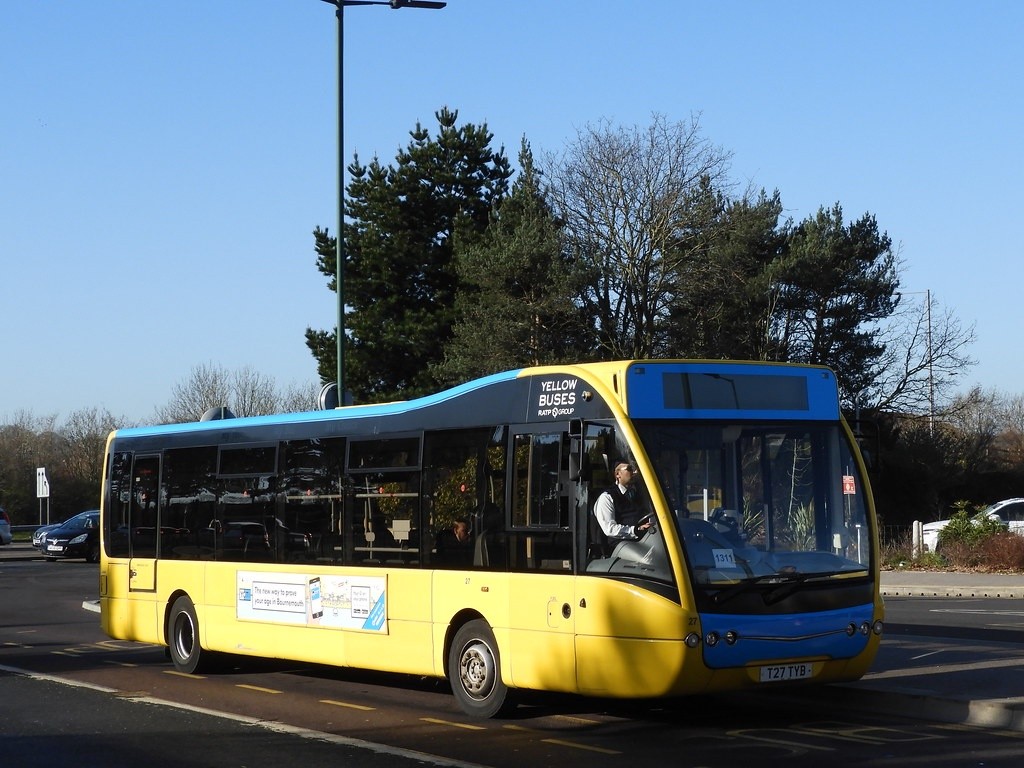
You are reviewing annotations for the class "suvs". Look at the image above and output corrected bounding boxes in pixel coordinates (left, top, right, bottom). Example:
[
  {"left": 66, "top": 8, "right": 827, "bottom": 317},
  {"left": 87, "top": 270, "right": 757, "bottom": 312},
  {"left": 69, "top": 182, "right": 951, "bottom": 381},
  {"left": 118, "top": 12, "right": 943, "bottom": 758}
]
[
  {"left": 0, "top": 509, "right": 13, "bottom": 547},
  {"left": 923, "top": 498, "right": 1024, "bottom": 557}
]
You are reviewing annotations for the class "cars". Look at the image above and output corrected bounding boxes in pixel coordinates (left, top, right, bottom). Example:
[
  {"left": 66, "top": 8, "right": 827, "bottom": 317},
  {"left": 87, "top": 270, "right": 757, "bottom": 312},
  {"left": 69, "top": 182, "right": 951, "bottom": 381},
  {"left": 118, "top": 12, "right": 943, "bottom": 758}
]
[
  {"left": 111, "top": 520, "right": 314, "bottom": 560},
  {"left": 32, "top": 519, "right": 93, "bottom": 548},
  {"left": 39, "top": 513, "right": 102, "bottom": 564}
]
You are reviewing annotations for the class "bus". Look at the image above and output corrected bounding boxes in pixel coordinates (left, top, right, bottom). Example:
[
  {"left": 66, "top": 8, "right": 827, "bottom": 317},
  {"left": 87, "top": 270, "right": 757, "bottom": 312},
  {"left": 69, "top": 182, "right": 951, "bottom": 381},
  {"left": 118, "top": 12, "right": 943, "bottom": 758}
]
[{"left": 97, "top": 357, "right": 886, "bottom": 723}]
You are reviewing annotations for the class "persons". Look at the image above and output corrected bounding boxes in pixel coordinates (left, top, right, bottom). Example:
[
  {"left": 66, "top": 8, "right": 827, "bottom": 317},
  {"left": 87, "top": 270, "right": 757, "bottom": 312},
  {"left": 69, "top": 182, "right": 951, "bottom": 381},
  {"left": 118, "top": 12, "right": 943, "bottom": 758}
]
[
  {"left": 437, "top": 516, "right": 472, "bottom": 555},
  {"left": 593, "top": 458, "right": 658, "bottom": 557}
]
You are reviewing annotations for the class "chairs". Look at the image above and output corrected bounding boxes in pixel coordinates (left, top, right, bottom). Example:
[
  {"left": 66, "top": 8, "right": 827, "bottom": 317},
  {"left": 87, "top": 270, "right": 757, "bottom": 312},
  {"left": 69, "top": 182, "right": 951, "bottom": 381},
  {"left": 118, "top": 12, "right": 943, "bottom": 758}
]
[{"left": 132, "top": 516, "right": 316, "bottom": 562}]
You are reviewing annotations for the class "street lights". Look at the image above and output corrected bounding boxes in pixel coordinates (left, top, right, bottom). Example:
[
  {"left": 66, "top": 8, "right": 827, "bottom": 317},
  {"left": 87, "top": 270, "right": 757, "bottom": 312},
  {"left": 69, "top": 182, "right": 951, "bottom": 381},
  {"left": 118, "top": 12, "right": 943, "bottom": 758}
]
[{"left": 328, "top": 0, "right": 447, "bottom": 419}]
